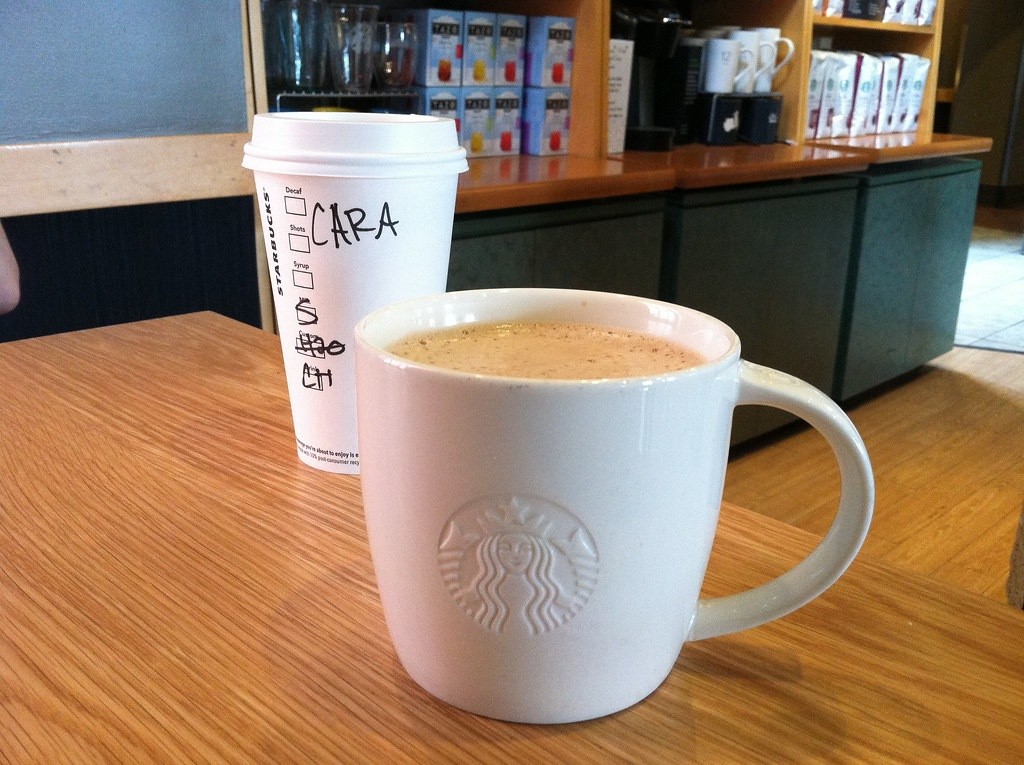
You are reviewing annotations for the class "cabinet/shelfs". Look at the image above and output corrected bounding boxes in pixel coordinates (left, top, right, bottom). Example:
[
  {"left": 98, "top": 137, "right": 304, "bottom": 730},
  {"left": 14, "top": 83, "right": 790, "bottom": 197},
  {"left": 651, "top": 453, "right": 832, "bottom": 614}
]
[{"left": 236, "top": 0, "right": 995, "bottom": 446}]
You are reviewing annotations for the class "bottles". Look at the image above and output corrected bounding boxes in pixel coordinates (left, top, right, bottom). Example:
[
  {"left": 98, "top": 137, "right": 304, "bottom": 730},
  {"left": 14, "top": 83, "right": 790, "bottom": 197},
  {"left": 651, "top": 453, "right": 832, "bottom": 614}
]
[
  {"left": 552, "top": 40, "right": 565, "bottom": 84},
  {"left": 471, "top": 36, "right": 487, "bottom": 80},
  {"left": 471, "top": 109, "right": 484, "bottom": 152},
  {"left": 504, "top": 37, "right": 518, "bottom": 82},
  {"left": 500, "top": 109, "right": 515, "bottom": 151},
  {"left": 550, "top": 110, "right": 562, "bottom": 151},
  {"left": 439, "top": 34, "right": 453, "bottom": 82}
]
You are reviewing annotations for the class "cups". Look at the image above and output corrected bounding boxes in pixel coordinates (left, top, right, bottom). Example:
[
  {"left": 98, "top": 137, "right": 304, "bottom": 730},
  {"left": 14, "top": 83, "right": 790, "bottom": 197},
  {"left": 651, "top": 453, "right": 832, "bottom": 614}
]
[
  {"left": 679, "top": 24, "right": 797, "bottom": 93},
  {"left": 241, "top": 111, "right": 470, "bottom": 474},
  {"left": 267, "top": 0, "right": 417, "bottom": 93},
  {"left": 356, "top": 288, "right": 875, "bottom": 726}
]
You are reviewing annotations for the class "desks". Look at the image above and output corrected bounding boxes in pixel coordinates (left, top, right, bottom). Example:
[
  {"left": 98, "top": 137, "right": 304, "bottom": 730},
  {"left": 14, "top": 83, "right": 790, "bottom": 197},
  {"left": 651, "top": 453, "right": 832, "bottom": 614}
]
[{"left": 0, "top": 312, "right": 1024, "bottom": 765}]
